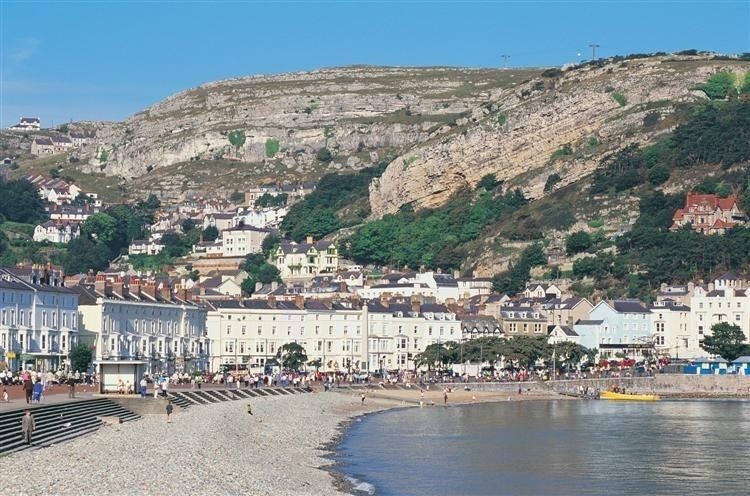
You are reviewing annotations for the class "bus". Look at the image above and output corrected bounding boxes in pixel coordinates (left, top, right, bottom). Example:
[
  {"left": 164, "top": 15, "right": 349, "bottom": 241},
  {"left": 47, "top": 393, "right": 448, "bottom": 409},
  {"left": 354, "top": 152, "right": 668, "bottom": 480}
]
[{"left": 218, "top": 363, "right": 264, "bottom": 375}]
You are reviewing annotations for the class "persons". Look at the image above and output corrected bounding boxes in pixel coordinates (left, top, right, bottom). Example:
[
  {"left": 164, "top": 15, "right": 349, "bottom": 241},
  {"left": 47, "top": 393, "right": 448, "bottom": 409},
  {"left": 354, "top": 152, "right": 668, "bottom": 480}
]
[
  {"left": 166, "top": 401, "right": 173, "bottom": 423},
  {"left": 738, "top": 366, "right": 745, "bottom": 375},
  {"left": 22, "top": 410, "right": 36, "bottom": 444}
]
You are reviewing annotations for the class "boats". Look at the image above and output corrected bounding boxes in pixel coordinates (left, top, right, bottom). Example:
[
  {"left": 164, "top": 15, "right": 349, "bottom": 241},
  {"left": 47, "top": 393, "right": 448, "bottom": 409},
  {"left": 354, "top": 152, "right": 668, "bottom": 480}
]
[{"left": 600, "top": 391, "right": 661, "bottom": 401}]
